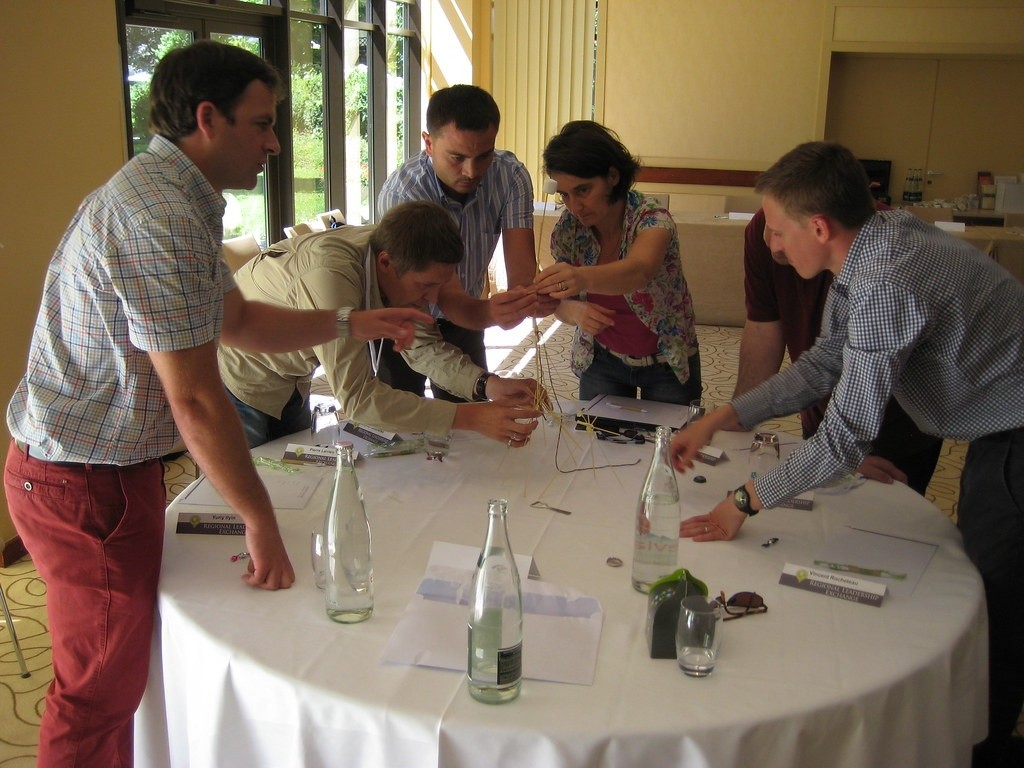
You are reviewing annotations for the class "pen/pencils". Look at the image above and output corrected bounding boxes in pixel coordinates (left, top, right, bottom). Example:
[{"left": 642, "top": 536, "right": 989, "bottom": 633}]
[
  {"left": 606, "top": 402, "right": 648, "bottom": 413},
  {"left": 416, "top": 439, "right": 449, "bottom": 449},
  {"left": 365, "top": 450, "right": 415, "bottom": 457},
  {"left": 814, "top": 560, "right": 906, "bottom": 581}
]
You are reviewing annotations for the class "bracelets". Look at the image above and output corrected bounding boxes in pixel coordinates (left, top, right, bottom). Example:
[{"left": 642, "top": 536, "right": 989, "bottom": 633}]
[
  {"left": 476, "top": 372, "right": 500, "bottom": 399},
  {"left": 336, "top": 307, "right": 355, "bottom": 339}
]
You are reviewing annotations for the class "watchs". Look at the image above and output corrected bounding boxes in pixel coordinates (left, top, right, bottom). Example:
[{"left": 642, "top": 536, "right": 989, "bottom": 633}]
[{"left": 733, "top": 484, "right": 759, "bottom": 517}]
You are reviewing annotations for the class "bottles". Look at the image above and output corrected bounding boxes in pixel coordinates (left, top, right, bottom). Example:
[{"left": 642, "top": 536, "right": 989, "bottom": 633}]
[
  {"left": 918, "top": 169, "right": 923, "bottom": 202},
  {"left": 909, "top": 169, "right": 918, "bottom": 202},
  {"left": 467, "top": 499, "right": 523, "bottom": 704},
  {"left": 903, "top": 168, "right": 912, "bottom": 202},
  {"left": 632, "top": 426, "right": 681, "bottom": 593},
  {"left": 325, "top": 440, "right": 375, "bottom": 624}
]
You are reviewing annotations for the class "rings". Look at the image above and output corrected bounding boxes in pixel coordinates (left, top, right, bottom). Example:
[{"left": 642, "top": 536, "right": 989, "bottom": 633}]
[
  {"left": 506, "top": 440, "right": 511, "bottom": 448},
  {"left": 705, "top": 526, "right": 709, "bottom": 532},
  {"left": 511, "top": 432, "right": 517, "bottom": 440},
  {"left": 559, "top": 282, "right": 564, "bottom": 290}
]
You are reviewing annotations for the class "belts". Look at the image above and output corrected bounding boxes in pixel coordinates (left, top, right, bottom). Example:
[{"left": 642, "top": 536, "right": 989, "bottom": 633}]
[
  {"left": 594, "top": 336, "right": 698, "bottom": 366},
  {"left": 16, "top": 440, "right": 117, "bottom": 468}
]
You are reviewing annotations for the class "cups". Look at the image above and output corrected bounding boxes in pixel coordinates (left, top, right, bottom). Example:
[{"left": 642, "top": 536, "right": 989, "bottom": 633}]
[
  {"left": 311, "top": 528, "right": 325, "bottom": 589},
  {"left": 423, "top": 430, "right": 454, "bottom": 462},
  {"left": 676, "top": 595, "right": 725, "bottom": 678},
  {"left": 750, "top": 433, "right": 780, "bottom": 479},
  {"left": 687, "top": 399, "right": 715, "bottom": 446},
  {"left": 311, "top": 404, "right": 340, "bottom": 449}
]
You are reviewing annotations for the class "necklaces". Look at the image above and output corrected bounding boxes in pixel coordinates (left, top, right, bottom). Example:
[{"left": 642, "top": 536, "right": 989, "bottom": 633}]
[{"left": 601, "top": 226, "right": 623, "bottom": 255}]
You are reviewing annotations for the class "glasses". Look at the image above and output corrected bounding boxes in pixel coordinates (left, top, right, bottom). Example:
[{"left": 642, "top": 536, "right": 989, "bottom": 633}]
[
  {"left": 710, "top": 591, "right": 768, "bottom": 622},
  {"left": 749, "top": 432, "right": 780, "bottom": 458}
]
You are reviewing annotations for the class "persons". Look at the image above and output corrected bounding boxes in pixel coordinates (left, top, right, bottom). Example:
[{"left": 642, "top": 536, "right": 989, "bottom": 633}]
[
  {"left": 217, "top": 199, "right": 554, "bottom": 449},
  {"left": 377, "top": 83, "right": 561, "bottom": 402},
  {"left": 664, "top": 142, "right": 1024, "bottom": 767},
  {"left": 4, "top": 40, "right": 435, "bottom": 768},
  {"left": 536, "top": 121, "right": 701, "bottom": 408}
]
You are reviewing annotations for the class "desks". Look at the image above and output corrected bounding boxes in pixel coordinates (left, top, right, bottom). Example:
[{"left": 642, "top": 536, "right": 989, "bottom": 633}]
[
  {"left": 134, "top": 394, "right": 990, "bottom": 768},
  {"left": 533, "top": 211, "right": 1024, "bottom": 329}
]
